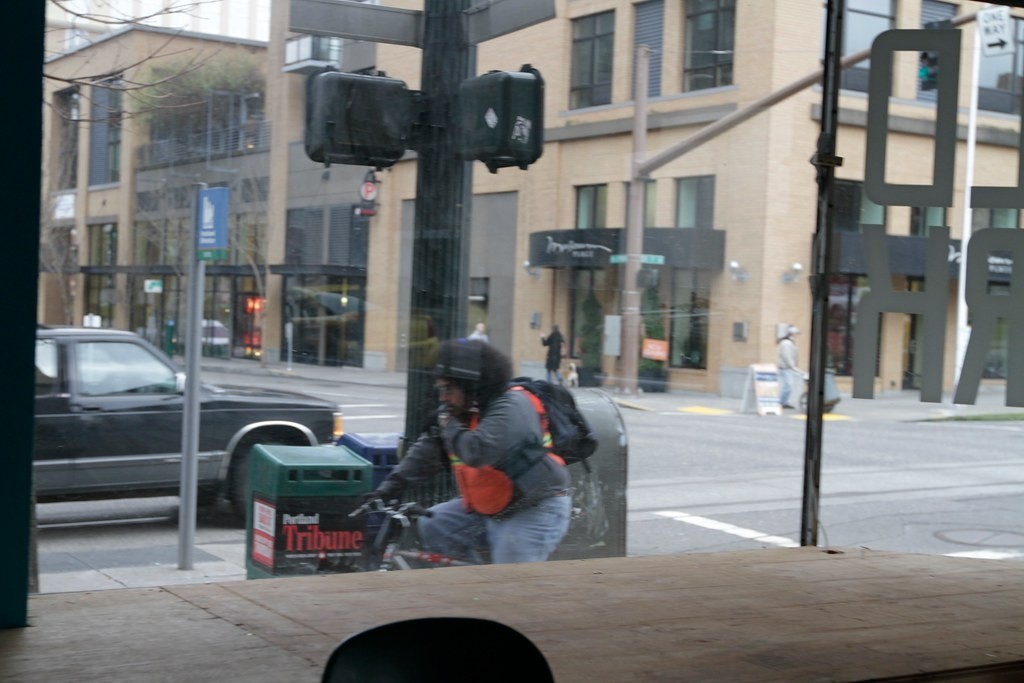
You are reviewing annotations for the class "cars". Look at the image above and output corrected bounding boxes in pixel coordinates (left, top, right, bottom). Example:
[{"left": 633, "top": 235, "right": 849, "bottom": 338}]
[
  {"left": 284, "top": 287, "right": 438, "bottom": 361},
  {"left": 35, "top": 327, "right": 343, "bottom": 534},
  {"left": 202, "top": 319, "right": 230, "bottom": 348}
]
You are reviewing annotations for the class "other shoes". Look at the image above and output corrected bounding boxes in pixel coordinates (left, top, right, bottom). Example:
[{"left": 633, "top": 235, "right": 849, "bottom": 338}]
[
  {"left": 783, "top": 405, "right": 794, "bottom": 409},
  {"left": 559, "top": 378, "right": 562, "bottom": 385}
]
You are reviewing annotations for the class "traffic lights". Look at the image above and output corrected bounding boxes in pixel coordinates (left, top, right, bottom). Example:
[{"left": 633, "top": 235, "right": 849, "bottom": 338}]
[{"left": 918, "top": 49, "right": 938, "bottom": 90}]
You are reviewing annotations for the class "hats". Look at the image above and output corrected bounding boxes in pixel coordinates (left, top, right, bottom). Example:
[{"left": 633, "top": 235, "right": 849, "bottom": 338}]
[
  {"left": 424, "top": 343, "right": 483, "bottom": 380},
  {"left": 788, "top": 326, "right": 801, "bottom": 334}
]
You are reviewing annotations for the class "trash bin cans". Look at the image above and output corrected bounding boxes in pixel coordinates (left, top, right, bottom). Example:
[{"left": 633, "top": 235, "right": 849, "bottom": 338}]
[
  {"left": 337, "top": 431, "right": 404, "bottom": 487},
  {"left": 244, "top": 443, "right": 375, "bottom": 583},
  {"left": 545, "top": 386, "right": 630, "bottom": 561}
]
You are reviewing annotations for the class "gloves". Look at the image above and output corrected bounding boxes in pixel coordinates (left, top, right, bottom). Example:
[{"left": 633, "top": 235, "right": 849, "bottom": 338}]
[
  {"left": 357, "top": 492, "right": 388, "bottom": 507},
  {"left": 416, "top": 413, "right": 437, "bottom": 434}
]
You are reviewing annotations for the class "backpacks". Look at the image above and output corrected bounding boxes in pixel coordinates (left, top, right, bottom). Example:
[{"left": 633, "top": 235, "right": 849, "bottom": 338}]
[{"left": 487, "top": 377, "right": 599, "bottom": 465}]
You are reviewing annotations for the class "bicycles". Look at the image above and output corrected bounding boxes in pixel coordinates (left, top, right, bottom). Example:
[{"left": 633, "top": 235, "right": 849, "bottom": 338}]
[{"left": 316, "top": 496, "right": 494, "bottom": 572}]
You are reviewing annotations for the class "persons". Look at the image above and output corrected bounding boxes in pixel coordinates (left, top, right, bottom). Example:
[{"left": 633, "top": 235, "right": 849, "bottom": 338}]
[
  {"left": 468, "top": 323, "right": 489, "bottom": 342},
  {"left": 776, "top": 326, "right": 801, "bottom": 409},
  {"left": 372, "top": 338, "right": 572, "bottom": 563},
  {"left": 541, "top": 325, "right": 565, "bottom": 383}
]
